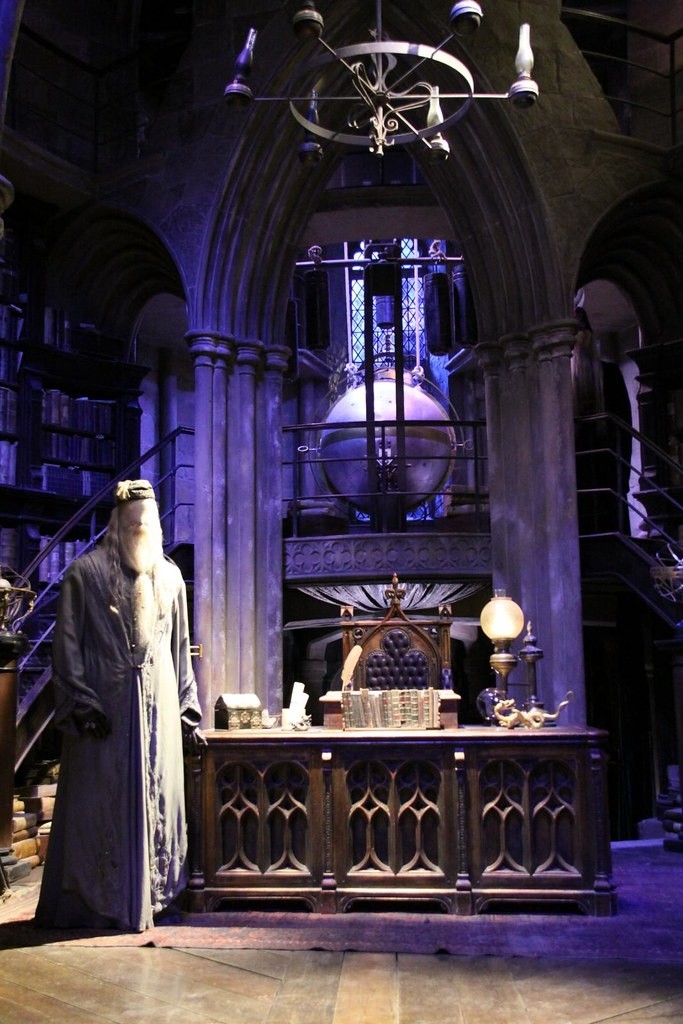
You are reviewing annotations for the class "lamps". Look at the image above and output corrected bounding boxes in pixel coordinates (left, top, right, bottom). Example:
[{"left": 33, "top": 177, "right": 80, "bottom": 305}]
[
  {"left": 479, "top": 588, "right": 525, "bottom": 699},
  {"left": 223, "top": 0, "right": 538, "bottom": 166}
]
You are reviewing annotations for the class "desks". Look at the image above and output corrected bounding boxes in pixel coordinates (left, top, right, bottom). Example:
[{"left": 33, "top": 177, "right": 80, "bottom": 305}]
[
  {"left": 319, "top": 690, "right": 461, "bottom": 728},
  {"left": 184, "top": 722, "right": 618, "bottom": 918}
]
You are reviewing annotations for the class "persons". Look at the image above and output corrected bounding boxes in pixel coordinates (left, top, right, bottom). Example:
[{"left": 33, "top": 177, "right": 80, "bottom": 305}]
[{"left": 34, "top": 480, "right": 207, "bottom": 929}]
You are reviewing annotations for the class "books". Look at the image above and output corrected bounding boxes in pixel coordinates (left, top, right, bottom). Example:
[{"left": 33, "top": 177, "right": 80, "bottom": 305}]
[
  {"left": 0, "top": 229, "right": 119, "bottom": 584},
  {"left": 342, "top": 689, "right": 439, "bottom": 728}
]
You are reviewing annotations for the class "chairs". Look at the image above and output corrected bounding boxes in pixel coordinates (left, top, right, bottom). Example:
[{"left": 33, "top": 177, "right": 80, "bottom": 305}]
[{"left": 340, "top": 572, "right": 453, "bottom": 692}]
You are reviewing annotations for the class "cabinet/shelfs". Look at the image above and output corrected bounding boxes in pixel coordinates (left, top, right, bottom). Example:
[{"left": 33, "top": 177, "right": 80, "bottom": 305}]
[{"left": 0, "top": 189, "right": 152, "bottom": 703}]
